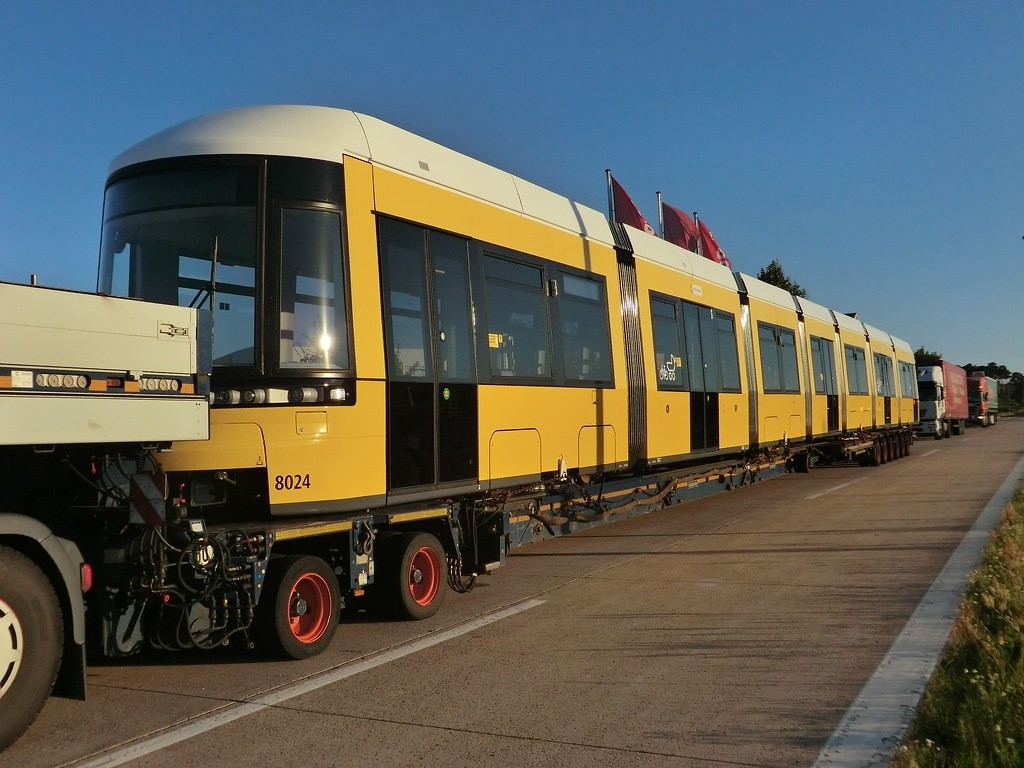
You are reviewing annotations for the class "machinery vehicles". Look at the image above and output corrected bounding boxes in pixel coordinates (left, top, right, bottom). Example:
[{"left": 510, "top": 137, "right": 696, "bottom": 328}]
[{"left": 0, "top": 281, "right": 210, "bottom": 750}]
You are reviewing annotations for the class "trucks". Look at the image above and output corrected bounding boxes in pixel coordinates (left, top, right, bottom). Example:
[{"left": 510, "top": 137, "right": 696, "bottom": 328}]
[
  {"left": 966, "top": 377, "right": 999, "bottom": 429},
  {"left": 909, "top": 360, "right": 969, "bottom": 441}
]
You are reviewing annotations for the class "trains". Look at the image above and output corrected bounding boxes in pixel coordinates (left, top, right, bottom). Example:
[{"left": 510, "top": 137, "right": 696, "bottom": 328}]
[{"left": 87, "top": 102, "right": 924, "bottom": 661}]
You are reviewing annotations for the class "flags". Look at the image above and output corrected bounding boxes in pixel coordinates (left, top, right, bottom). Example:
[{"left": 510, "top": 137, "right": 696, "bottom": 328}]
[
  {"left": 612, "top": 176, "right": 658, "bottom": 238},
  {"left": 662, "top": 202, "right": 700, "bottom": 254},
  {"left": 697, "top": 219, "right": 732, "bottom": 270}
]
